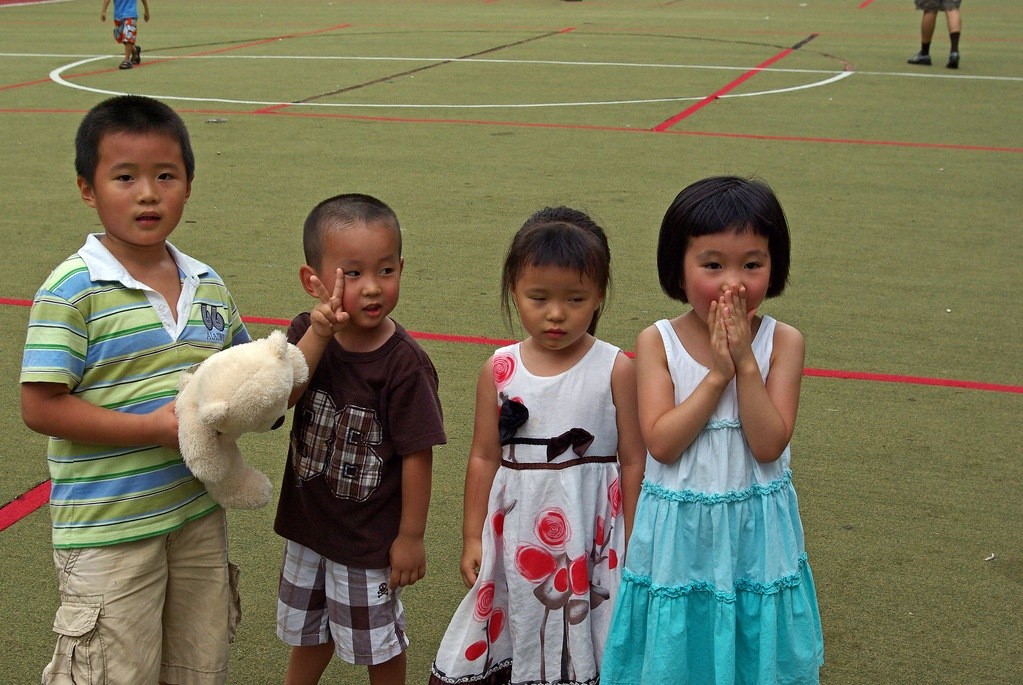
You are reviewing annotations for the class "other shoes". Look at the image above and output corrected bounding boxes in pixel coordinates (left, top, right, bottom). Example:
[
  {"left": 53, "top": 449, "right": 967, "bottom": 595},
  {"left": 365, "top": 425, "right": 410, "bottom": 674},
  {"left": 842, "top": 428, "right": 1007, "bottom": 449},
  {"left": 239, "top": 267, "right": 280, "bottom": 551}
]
[
  {"left": 946, "top": 52, "right": 959, "bottom": 69},
  {"left": 908, "top": 53, "right": 931, "bottom": 64}
]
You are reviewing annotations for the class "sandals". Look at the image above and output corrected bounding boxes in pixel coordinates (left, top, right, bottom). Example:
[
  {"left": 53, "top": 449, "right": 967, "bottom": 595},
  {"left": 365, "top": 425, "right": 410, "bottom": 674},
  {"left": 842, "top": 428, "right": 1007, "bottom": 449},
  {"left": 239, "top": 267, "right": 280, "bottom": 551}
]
[
  {"left": 120, "top": 61, "right": 132, "bottom": 69},
  {"left": 131, "top": 46, "right": 140, "bottom": 64}
]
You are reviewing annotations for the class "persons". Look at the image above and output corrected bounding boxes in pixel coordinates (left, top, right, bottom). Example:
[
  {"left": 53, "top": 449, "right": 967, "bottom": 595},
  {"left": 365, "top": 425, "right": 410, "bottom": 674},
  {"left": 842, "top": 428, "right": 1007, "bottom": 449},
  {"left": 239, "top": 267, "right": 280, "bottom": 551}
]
[
  {"left": 19, "top": 95, "right": 255, "bottom": 685},
  {"left": 276, "top": 191, "right": 449, "bottom": 685},
  {"left": 909, "top": 0, "right": 963, "bottom": 68},
  {"left": 101, "top": 1, "right": 151, "bottom": 71},
  {"left": 602, "top": 176, "right": 824, "bottom": 685},
  {"left": 425, "top": 207, "right": 644, "bottom": 685}
]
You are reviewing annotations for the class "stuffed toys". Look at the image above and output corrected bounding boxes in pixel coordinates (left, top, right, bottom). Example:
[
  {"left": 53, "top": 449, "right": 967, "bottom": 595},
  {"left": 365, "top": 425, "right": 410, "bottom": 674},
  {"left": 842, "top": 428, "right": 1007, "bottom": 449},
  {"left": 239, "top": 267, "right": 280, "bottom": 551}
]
[{"left": 176, "top": 330, "right": 308, "bottom": 512}]
